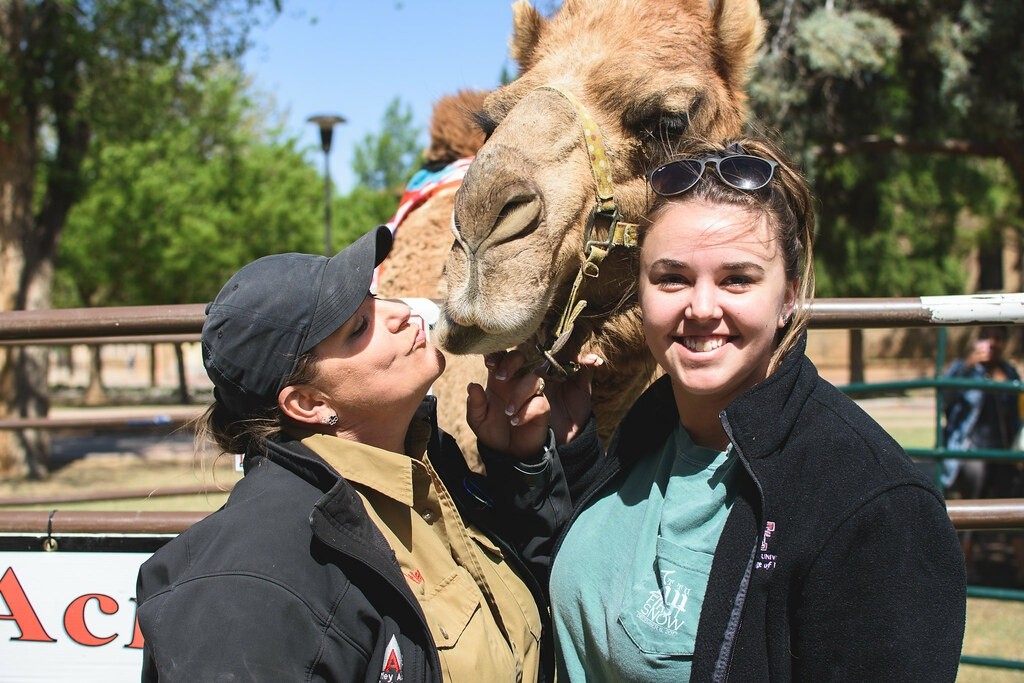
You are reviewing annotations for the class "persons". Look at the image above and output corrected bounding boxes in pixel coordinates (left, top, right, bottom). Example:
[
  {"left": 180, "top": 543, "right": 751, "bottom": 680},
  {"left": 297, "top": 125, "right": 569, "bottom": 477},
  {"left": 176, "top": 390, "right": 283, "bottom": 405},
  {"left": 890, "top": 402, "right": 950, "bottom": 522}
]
[
  {"left": 524, "top": 135, "right": 968, "bottom": 683},
  {"left": 940, "top": 323, "right": 1023, "bottom": 559},
  {"left": 135, "top": 220, "right": 574, "bottom": 683}
]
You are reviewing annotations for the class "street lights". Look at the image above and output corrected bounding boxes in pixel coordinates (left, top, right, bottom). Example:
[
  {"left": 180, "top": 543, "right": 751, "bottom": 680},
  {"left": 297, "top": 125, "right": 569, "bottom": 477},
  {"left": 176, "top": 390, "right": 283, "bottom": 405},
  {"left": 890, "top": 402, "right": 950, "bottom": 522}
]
[{"left": 308, "top": 116, "right": 350, "bottom": 258}]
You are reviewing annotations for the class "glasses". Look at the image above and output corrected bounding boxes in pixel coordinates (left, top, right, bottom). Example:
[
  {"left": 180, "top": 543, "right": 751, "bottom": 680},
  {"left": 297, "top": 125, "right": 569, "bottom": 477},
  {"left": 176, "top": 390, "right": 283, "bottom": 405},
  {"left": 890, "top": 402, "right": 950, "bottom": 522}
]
[{"left": 645, "top": 154, "right": 781, "bottom": 196}]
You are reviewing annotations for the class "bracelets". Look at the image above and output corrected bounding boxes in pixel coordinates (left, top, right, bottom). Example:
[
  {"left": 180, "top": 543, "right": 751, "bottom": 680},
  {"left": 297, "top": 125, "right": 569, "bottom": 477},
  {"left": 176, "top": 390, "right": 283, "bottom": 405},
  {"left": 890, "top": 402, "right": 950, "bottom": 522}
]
[{"left": 513, "top": 446, "right": 552, "bottom": 476}]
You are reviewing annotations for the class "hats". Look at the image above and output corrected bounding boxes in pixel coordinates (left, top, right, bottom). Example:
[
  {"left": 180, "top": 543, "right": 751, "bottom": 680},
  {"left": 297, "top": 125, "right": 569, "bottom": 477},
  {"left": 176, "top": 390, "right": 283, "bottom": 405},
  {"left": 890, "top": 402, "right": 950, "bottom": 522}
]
[{"left": 201, "top": 225, "right": 394, "bottom": 412}]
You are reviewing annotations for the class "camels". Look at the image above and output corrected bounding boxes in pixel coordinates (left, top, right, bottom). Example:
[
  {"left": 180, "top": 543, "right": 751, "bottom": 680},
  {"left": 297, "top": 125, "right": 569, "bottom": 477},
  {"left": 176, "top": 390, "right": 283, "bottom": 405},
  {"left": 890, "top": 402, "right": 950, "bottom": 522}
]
[
  {"left": 938, "top": 218, "right": 1024, "bottom": 497},
  {"left": 372, "top": 1, "right": 772, "bottom": 475}
]
